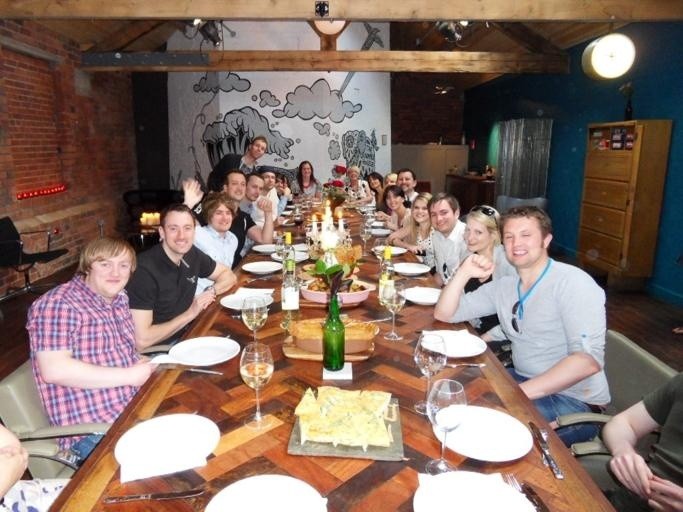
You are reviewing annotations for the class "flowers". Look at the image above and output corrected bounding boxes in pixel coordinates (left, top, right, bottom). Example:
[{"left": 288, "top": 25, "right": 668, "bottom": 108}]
[{"left": 323, "top": 165, "right": 347, "bottom": 192}]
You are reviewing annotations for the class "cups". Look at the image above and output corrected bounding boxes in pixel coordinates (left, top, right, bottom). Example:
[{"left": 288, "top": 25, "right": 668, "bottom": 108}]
[
  {"left": 301, "top": 199, "right": 312, "bottom": 212},
  {"left": 363, "top": 214, "right": 375, "bottom": 230}
]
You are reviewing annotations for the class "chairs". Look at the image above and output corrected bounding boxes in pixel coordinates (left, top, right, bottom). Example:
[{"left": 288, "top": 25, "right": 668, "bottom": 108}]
[
  {"left": 494, "top": 346, "right": 512, "bottom": 359},
  {"left": 568, "top": 440, "right": 612, "bottom": 462},
  {"left": 0, "top": 342, "right": 174, "bottom": 482},
  {"left": 120, "top": 187, "right": 182, "bottom": 220},
  {"left": 0, "top": 217, "right": 69, "bottom": 298},
  {"left": 501, "top": 326, "right": 678, "bottom": 462}
]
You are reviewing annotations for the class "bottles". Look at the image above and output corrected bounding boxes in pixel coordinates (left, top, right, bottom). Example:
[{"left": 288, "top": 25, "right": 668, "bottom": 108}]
[
  {"left": 375, "top": 241, "right": 396, "bottom": 304},
  {"left": 278, "top": 259, "right": 299, "bottom": 329},
  {"left": 460, "top": 130, "right": 465, "bottom": 145},
  {"left": 320, "top": 293, "right": 346, "bottom": 372}
]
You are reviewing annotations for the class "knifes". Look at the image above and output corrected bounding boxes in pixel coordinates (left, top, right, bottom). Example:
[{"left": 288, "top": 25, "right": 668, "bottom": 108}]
[
  {"left": 99, "top": 485, "right": 205, "bottom": 502},
  {"left": 158, "top": 363, "right": 222, "bottom": 376},
  {"left": 439, "top": 360, "right": 485, "bottom": 370},
  {"left": 527, "top": 419, "right": 563, "bottom": 480}
]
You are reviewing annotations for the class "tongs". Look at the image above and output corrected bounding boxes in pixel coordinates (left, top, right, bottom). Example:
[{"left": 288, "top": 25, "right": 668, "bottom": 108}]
[{"left": 236, "top": 343, "right": 277, "bottom": 431}]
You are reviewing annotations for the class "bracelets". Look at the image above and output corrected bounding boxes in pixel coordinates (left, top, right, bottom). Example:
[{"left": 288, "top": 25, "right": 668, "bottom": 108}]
[{"left": 203, "top": 285, "right": 217, "bottom": 296}]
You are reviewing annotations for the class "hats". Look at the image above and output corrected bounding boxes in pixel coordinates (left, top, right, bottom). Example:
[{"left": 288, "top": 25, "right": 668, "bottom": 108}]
[{"left": 257, "top": 165, "right": 276, "bottom": 177}]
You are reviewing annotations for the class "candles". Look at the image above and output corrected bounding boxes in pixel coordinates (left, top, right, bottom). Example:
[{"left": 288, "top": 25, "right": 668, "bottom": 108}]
[{"left": 309, "top": 200, "right": 344, "bottom": 234}]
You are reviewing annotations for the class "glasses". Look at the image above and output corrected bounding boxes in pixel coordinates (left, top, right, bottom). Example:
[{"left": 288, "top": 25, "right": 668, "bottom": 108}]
[
  {"left": 471, "top": 204, "right": 499, "bottom": 231},
  {"left": 512, "top": 301, "right": 520, "bottom": 332}
]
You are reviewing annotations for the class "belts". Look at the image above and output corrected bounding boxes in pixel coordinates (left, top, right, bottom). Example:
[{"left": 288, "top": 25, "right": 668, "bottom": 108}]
[{"left": 584, "top": 402, "right": 601, "bottom": 413}]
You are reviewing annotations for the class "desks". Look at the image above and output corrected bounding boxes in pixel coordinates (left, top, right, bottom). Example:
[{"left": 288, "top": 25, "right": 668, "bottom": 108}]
[{"left": 112, "top": 224, "right": 161, "bottom": 252}]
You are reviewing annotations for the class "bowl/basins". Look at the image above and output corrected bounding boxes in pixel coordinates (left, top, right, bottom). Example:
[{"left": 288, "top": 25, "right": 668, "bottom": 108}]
[{"left": 288, "top": 316, "right": 380, "bottom": 354}]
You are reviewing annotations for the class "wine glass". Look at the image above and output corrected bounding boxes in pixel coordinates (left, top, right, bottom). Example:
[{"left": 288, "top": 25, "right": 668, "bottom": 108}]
[
  {"left": 380, "top": 280, "right": 406, "bottom": 340},
  {"left": 240, "top": 296, "right": 267, "bottom": 343},
  {"left": 293, "top": 212, "right": 304, "bottom": 240},
  {"left": 358, "top": 223, "right": 372, "bottom": 256},
  {"left": 422, "top": 377, "right": 469, "bottom": 475},
  {"left": 408, "top": 333, "right": 447, "bottom": 417}
]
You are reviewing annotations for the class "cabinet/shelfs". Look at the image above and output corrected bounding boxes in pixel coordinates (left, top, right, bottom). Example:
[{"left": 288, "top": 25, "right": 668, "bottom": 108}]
[
  {"left": 392, "top": 142, "right": 471, "bottom": 195},
  {"left": 444, "top": 170, "right": 495, "bottom": 216},
  {"left": 574, "top": 118, "right": 674, "bottom": 293}
]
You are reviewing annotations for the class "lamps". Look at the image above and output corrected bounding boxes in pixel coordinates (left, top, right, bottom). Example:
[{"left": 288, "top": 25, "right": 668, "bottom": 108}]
[
  {"left": 578, "top": 20, "right": 638, "bottom": 83},
  {"left": 182, "top": 18, "right": 223, "bottom": 47}
]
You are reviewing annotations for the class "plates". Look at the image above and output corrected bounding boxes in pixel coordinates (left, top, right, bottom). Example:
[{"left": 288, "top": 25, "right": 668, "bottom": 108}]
[
  {"left": 290, "top": 243, "right": 308, "bottom": 251},
  {"left": 368, "top": 229, "right": 391, "bottom": 237},
  {"left": 112, "top": 411, "right": 221, "bottom": 473},
  {"left": 270, "top": 250, "right": 309, "bottom": 263},
  {"left": 297, "top": 277, "right": 376, "bottom": 304},
  {"left": 286, "top": 205, "right": 294, "bottom": 209},
  {"left": 250, "top": 244, "right": 284, "bottom": 255},
  {"left": 280, "top": 231, "right": 296, "bottom": 278},
  {"left": 240, "top": 260, "right": 282, "bottom": 276},
  {"left": 196, "top": 471, "right": 328, "bottom": 512},
  {"left": 279, "top": 219, "right": 295, "bottom": 227},
  {"left": 161, "top": 335, "right": 240, "bottom": 366},
  {"left": 398, "top": 286, "right": 441, "bottom": 306},
  {"left": 370, "top": 245, "right": 407, "bottom": 257},
  {"left": 219, "top": 291, "right": 273, "bottom": 310},
  {"left": 280, "top": 211, "right": 292, "bottom": 216},
  {"left": 431, "top": 404, "right": 534, "bottom": 463},
  {"left": 416, "top": 330, "right": 487, "bottom": 358},
  {"left": 272, "top": 230, "right": 285, "bottom": 243},
  {"left": 411, "top": 469, "right": 536, "bottom": 512},
  {"left": 369, "top": 221, "right": 384, "bottom": 228},
  {"left": 390, "top": 263, "right": 430, "bottom": 276}
]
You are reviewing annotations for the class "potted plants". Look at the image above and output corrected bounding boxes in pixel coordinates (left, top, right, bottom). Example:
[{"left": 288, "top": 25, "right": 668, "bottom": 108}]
[
  {"left": 303, "top": 258, "right": 370, "bottom": 369},
  {"left": 618, "top": 80, "right": 635, "bottom": 121}
]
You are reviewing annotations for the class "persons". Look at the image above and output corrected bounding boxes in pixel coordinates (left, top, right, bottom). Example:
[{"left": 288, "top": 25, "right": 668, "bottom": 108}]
[
  {"left": 25, "top": 233, "right": 155, "bottom": 465},
  {"left": 575, "top": 371, "right": 682, "bottom": 512},
  {"left": 0, "top": 418, "right": 72, "bottom": 511},
  {"left": 126, "top": 203, "right": 237, "bottom": 353},
  {"left": 426, "top": 193, "right": 477, "bottom": 286},
  {"left": 444, "top": 206, "right": 520, "bottom": 367},
  {"left": 433, "top": 205, "right": 612, "bottom": 448},
  {"left": 181, "top": 134, "right": 433, "bottom": 270}
]
